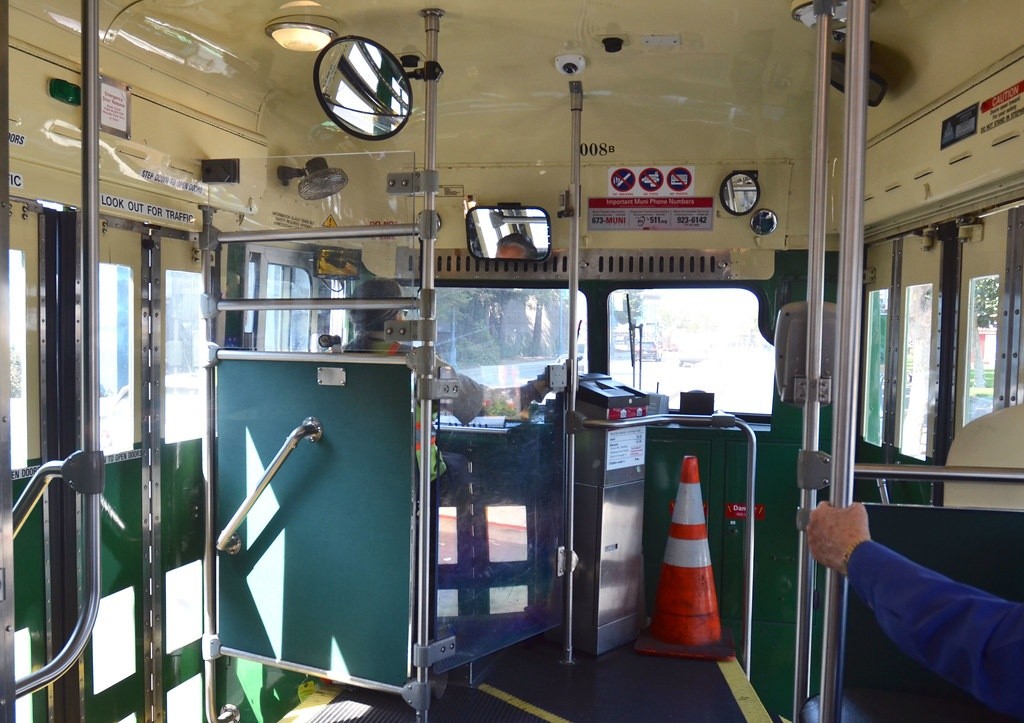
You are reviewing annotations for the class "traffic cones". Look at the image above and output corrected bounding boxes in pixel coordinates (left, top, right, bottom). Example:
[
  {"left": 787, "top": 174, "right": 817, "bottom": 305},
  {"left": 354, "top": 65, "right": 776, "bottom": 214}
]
[{"left": 630, "top": 452, "right": 737, "bottom": 663}]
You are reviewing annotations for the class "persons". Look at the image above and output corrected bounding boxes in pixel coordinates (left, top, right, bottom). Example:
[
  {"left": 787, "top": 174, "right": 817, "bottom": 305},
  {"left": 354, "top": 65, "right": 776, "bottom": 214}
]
[
  {"left": 324, "top": 276, "right": 556, "bottom": 483},
  {"left": 494, "top": 232, "right": 538, "bottom": 260},
  {"left": 805, "top": 500, "right": 1024, "bottom": 721}
]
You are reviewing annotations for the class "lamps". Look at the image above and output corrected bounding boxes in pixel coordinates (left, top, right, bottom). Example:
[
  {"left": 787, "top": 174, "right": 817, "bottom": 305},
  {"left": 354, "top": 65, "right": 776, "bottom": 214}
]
[{"left": 263, "top": 15, "right": 345, "bottom": 54}]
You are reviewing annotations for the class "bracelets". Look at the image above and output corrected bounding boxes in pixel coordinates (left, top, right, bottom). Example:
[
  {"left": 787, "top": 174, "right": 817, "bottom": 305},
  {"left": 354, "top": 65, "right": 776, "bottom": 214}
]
[{"left": 840, "top": 537, "right": 868, "bottom": 575}]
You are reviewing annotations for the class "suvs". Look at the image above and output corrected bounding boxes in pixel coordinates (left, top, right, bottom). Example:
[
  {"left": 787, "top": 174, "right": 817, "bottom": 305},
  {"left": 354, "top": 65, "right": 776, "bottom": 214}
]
[{"left": 633, "top": 341, "right": 663, "bottom": 362}]
